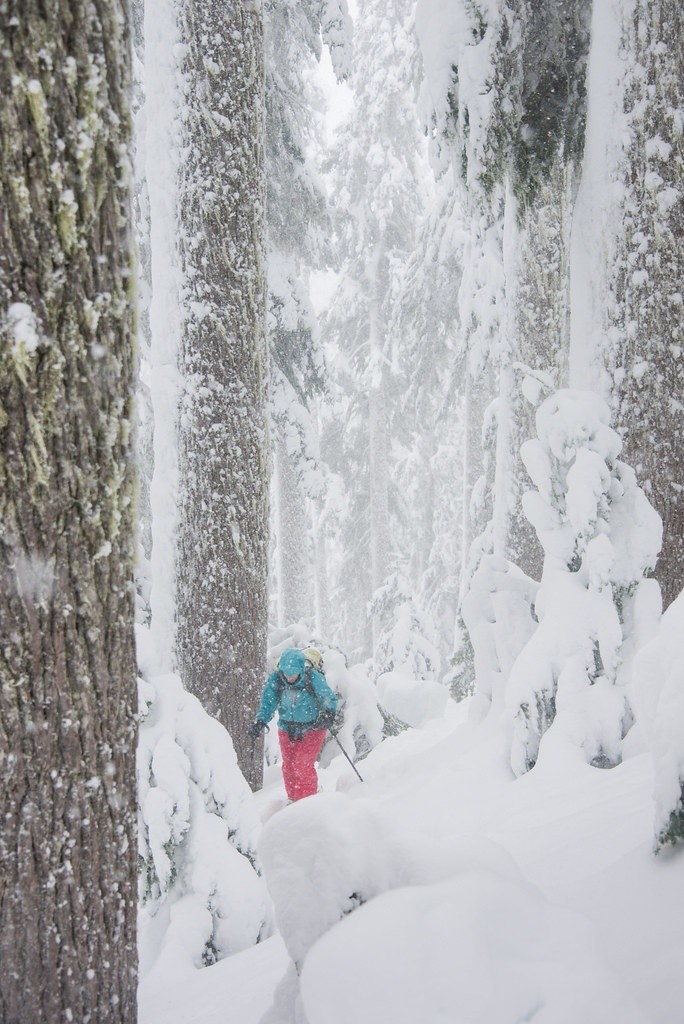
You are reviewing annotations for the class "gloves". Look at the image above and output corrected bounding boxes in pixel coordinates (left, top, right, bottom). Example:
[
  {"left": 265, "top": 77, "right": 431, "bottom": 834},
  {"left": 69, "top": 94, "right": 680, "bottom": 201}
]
[
  {"left": 314, "top": 709, "right": 336, "bottom": 730},
  {"left": 248, "top": 721, "right": 266, "bottom": 738}
]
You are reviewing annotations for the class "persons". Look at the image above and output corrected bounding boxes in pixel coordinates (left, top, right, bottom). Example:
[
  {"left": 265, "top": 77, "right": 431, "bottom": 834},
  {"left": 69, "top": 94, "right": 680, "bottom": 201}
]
[{"left": 245, "top": 648, "right": 339, "bottom": 804}]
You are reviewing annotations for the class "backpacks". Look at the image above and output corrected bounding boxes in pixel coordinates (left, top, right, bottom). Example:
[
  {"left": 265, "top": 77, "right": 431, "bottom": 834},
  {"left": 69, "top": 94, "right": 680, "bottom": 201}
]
[{"left": 278, "top": 647, "right": 324, "bottom": 710}]
